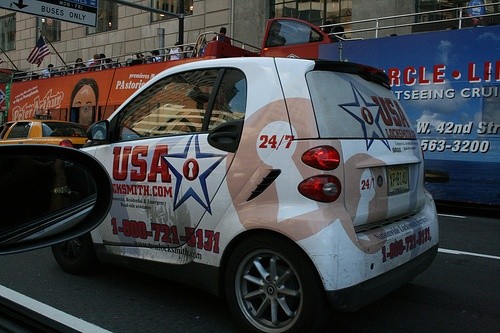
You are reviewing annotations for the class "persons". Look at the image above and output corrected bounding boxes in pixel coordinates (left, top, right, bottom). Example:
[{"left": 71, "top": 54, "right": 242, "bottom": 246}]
[
  {"left": 318, "top": 20, "right": 344, "bottom": 42},
  {"left": 39, "top": 27, "right": 230, "bottom": 81}
]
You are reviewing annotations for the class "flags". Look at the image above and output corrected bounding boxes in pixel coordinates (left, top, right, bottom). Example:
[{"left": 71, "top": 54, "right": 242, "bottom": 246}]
[{"left": 27, "top": 35, "right": 49, "bottom": 68}]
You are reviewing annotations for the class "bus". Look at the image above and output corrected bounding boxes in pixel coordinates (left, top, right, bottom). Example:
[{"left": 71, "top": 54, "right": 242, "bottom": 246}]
[
  {"left": 0, "top": 67, "right": 27, "bottom": 126},
  {"left": 259, "top": 1, "right": 499, "bottom": 207},
  {"left": 5, "top": 31, "right": 261, "bottom": 129}
]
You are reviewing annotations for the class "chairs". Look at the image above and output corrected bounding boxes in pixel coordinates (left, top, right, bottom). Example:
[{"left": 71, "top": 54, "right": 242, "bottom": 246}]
[{"left": 26, "top": 46, "right": 197, "bottom": 80}]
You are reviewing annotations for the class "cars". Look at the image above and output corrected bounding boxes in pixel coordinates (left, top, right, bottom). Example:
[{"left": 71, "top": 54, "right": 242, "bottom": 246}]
[
  {"left": 49, "top": 56, "right": 440, "bottom": 333},
  {"left": 0, "top": 114, "right": 92, "bottom": 150}
]
